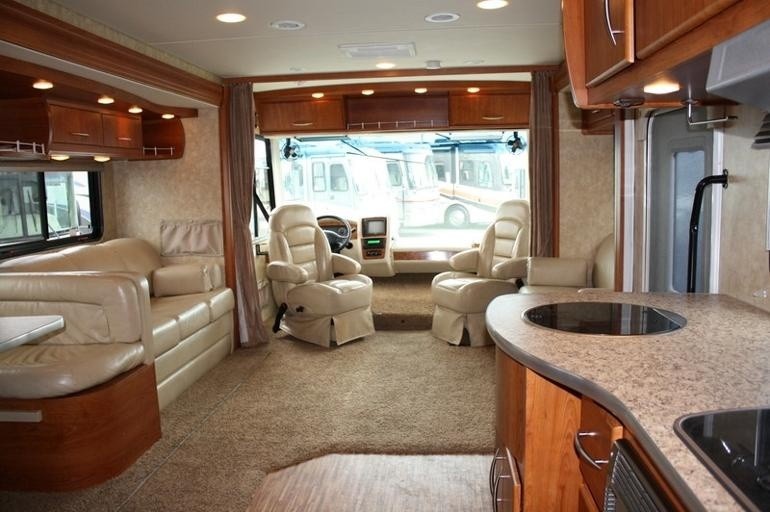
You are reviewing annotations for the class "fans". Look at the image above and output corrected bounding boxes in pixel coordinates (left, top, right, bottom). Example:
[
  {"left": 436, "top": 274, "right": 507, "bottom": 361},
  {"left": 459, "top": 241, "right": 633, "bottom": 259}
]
[
  {"left": 279, "top": 138, "right": 301, "bottom": 161},
  {"left": 503, "top": 131, "right": 527, "bottom": 155}
]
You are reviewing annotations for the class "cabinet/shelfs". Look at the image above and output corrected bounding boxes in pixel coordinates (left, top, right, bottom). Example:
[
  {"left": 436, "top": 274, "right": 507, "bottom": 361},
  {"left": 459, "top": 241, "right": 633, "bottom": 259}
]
[
  {"left": 1, "top": 98, "right": 178, "bottom": 159},
  {"left": 569, "top": 395, "right": 620, "bottom": 512},
  {"left": 485, "top": 442, "right": 522, "bottom": 512},
  {"left": 255, "top": 95, "right": 530, "bottom": 137},
  {"left": 581, "top": 0, "right": 770, "bottom": 106}
]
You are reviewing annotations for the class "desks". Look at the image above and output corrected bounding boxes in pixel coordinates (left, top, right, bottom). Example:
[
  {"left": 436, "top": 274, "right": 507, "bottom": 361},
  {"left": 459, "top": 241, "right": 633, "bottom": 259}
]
[{"left": 0, "top": 314, "right": 66, "bottom": 360}]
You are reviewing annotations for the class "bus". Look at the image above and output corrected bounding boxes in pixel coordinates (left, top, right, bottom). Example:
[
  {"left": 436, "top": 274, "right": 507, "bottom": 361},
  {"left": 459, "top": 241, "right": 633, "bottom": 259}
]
[
  {"left": 435, "top": 130, "right": 528, "bottom": 228},
  {"left": 363, "top": 136, "right": 443, "bottom": 230}
]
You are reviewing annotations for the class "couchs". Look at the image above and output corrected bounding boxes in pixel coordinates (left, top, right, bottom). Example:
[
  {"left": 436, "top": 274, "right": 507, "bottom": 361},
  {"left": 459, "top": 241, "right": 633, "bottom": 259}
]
[
  {"left": 0, "top": 273, "right": 162, "bottom": 492},
  {"left": 0, "top": 235, "right": 242, "bottom": 409}
]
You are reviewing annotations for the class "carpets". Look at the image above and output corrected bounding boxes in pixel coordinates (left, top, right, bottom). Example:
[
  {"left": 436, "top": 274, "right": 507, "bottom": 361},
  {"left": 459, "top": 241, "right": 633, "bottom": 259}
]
[{"left": 0, "top": 272, "right": 501, "bottom": 511}]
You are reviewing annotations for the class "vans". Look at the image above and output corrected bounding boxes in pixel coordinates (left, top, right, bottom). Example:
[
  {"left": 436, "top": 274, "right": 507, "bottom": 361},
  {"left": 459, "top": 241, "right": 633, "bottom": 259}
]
[{"left": 254, "top": 135, "right": 393, "bottom": 235}]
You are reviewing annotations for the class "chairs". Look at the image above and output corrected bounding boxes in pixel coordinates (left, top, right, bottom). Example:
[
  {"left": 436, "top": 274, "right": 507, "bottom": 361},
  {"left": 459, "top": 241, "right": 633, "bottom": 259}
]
[
  {"left": 429, "top": 198, "right": 529, "bottom": 349},
  {"left": 265, "top": 203, "right": 376, "bottom": 350}
]
